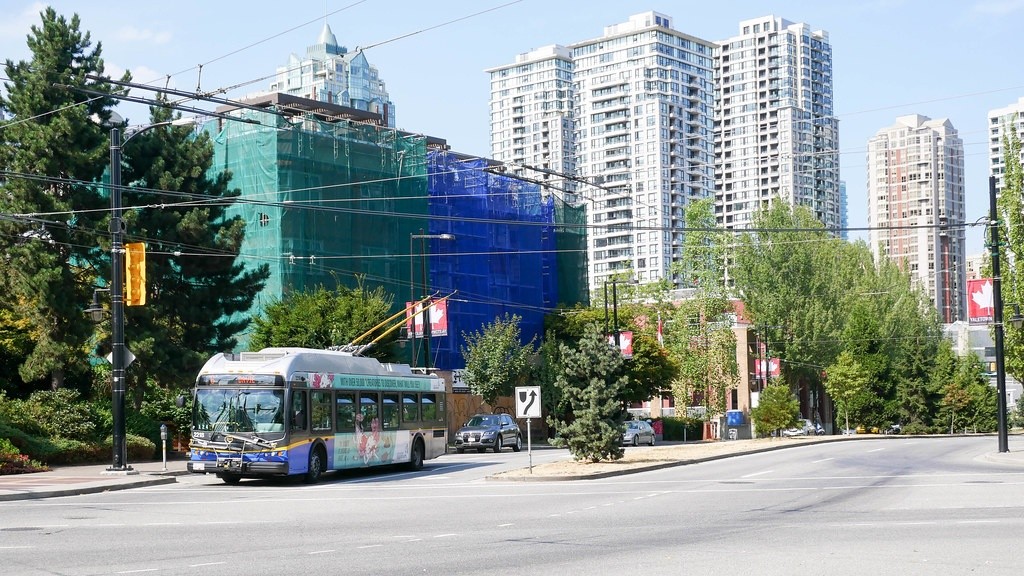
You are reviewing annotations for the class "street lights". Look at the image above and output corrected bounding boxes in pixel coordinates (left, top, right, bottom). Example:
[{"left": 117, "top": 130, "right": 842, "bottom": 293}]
[
  {"left": 604, "top": 279, "right": 639, "bottom": 339},
  {"left": 83, "top": 118, "right": 199, "bottom": 468},
  {"left": 410, "top": 232, "right": 455, "bottom": 370}
]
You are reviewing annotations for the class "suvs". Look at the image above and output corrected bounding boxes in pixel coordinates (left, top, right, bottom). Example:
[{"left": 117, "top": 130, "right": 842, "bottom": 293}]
[{"left": 453, "top": 413, "right": 522, "bottom": 453}]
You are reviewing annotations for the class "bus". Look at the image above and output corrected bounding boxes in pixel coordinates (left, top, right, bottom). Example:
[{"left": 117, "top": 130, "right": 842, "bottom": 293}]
[{"left": 177, "top": 347, "right": 445, "bottom": 486}]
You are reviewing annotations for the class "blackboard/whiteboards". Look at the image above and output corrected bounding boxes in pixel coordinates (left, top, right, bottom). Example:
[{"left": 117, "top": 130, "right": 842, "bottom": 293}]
[{"left": 728, "top": 429, "right": 737, "bottom": 439}]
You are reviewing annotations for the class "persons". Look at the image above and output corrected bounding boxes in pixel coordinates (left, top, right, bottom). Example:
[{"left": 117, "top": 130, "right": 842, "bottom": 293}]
[{"left": 353, "top": 414, "right": 381, "bottom": 465}]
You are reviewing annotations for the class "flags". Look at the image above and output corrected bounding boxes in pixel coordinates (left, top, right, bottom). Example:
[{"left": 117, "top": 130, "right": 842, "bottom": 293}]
[{"left": 657, "top": 317, "right": 664, "bottom": 347}]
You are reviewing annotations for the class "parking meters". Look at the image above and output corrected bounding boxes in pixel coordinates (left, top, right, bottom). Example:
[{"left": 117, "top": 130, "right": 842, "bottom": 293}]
[{"left": 161, "top": 424, "right": 167, "bottom": 471}]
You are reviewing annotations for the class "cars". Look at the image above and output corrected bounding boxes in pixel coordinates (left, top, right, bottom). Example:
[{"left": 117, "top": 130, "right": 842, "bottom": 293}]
[
  {"left": 884, "top": 425, "right": 902, "bottom": 435},
  {"left": 614, "top": 421, "right": 656, "bottom": 447},
  {"left": 856, "top": 423, "right": 880, "bottom": 434},
  {"left": 782, "top": 419, "right": 816, "bottom": 437}
]
